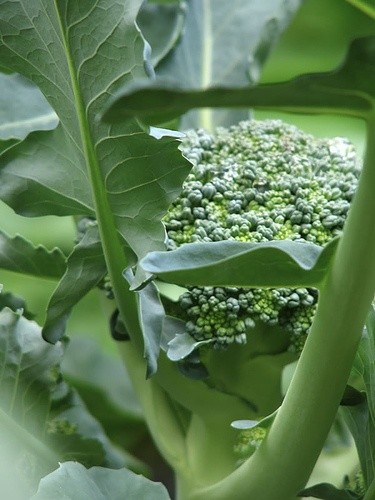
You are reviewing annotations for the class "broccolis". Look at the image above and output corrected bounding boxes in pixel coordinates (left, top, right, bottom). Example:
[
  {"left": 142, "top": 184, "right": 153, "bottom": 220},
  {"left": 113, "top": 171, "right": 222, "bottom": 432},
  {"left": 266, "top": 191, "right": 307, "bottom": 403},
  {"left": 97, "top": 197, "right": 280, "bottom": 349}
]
[{"left": 149, "top": 120, "right": 366, "bottom": 357}]
